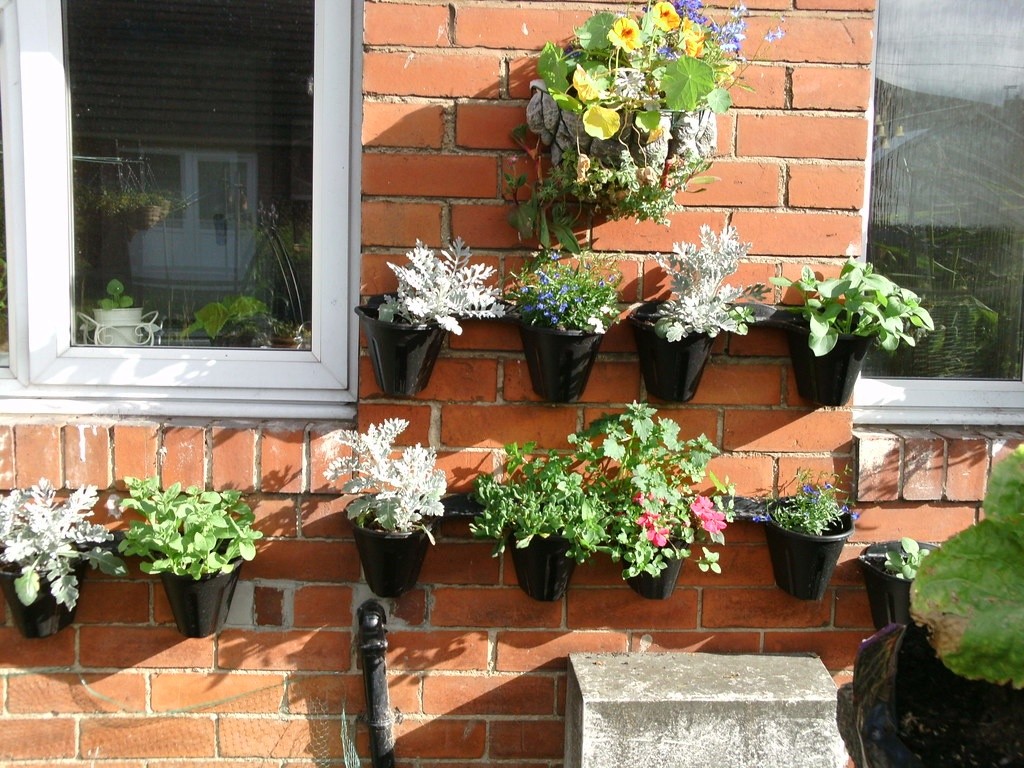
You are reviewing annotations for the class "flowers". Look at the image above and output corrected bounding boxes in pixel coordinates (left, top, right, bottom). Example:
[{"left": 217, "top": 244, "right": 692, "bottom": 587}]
[
  {"left": 567, "top": 400, "right": 738, "bottom": 580},
  {"left": 754, "top": 463, "right": 860, "bottom": 535},
  {"left": 504, "top": 250, "right": 626, "bottom": 336},
  {"left": 505, "top": 0, "right": 787, "bottom": 257}
]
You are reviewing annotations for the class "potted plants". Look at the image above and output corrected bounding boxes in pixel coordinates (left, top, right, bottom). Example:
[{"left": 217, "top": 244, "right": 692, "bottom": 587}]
[
  {"left": 0, "top": 476, "right": 127, "bottom": 638},
  {"left": 769, "top": 243, "right": 935, "bottom": 407},
  {"left": 179, "top": 296, "right": 306, "bottom": 348},
  {"left": 322, "top": 418, "right": 447, "bottom": 599},
  {"left": 858, "top": 537, "right": 941, "bottom": 631},
  {"left": 94, "top": 279, "right": 142, "bottom": 344},
  {"left": 854, "top": 444, "right": 1024, "bottom": 768},
  {"left": 118, "top": 475, "right": 263, "bottom": 637},
  {"left": 75, "top": 162, "right": 175, "bottom": 241},
  {"left": 355, "top": 235, "right": 507, "bottom": 396},
  {"left": 466, "top": 442, "right": 612, "bottom": 601},
  {"left": 630, "top": 223, "right": 773, "bottom": 402}
]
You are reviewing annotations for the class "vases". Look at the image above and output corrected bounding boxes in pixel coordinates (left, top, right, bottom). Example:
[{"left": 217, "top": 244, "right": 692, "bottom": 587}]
[
  {"left": 764, "top": 498, "right": 855, "bottom": 602},
  {"left": 526, "top": 79, "right": 719, "bottom": 167},
  {"left": 518, "top": 324, "right": 607, "bottom": 399},
  {"left": 619, "top": 539, "right": 689, "bottom": 598}
]
